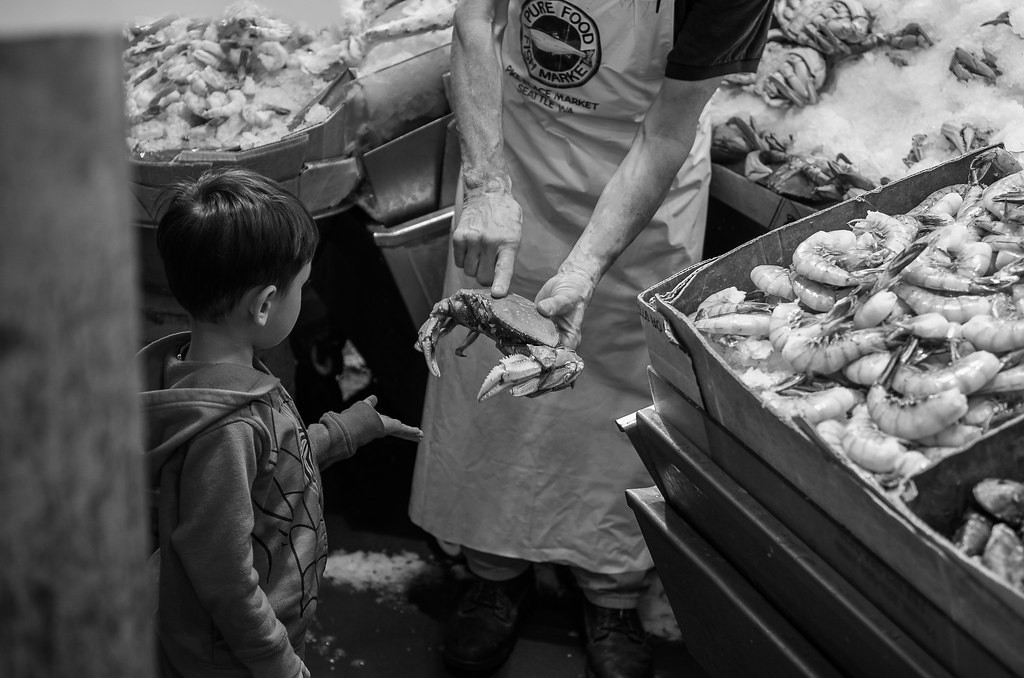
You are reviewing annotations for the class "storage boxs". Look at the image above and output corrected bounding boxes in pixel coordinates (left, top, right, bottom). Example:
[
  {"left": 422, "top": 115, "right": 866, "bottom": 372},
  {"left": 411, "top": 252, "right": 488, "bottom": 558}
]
[{"left": 636, "top": 141, "right": 1024, "bottom": 678}]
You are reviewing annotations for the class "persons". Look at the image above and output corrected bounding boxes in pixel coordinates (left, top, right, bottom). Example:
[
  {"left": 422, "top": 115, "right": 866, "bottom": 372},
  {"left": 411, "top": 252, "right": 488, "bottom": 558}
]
[
  {"left": 409, "top": 0, "right": 774, "bottom": 678},
  {"left": 134, "top": 168, "right": 425, "bottom": 678}
]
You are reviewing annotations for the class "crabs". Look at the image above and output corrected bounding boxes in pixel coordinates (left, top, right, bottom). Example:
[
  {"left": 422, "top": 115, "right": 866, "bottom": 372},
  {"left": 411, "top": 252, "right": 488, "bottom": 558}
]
[
  {"left": 415, "top": 286, "right": 585, "bottom": 401},
  {"left": 709, "top": 0, "right": 1023, "bottom": 205}
]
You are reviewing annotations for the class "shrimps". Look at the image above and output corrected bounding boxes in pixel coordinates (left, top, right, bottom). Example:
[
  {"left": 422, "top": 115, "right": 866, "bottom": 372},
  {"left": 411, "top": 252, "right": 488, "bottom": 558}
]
[
  {"left": 693, "top": 146, "right": 1023, "bottom": 592},
  {"left": 123, "top": 0, "right": 459, "bottom": 153}
]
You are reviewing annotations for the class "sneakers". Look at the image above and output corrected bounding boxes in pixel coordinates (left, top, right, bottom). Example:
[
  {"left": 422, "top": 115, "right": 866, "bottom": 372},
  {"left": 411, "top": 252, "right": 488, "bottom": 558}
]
[
  {"left": 442, "top": 565, "right": 532, "bottom": 674},
  {"left": 582, "top": 600, "right": 655, "bottom": 678}
]
